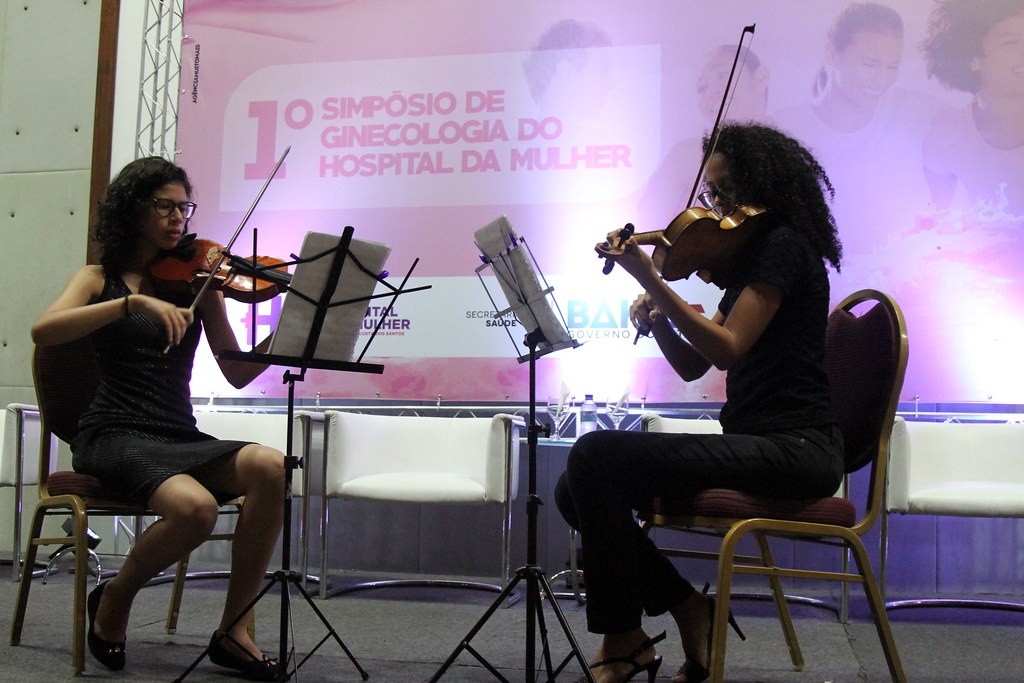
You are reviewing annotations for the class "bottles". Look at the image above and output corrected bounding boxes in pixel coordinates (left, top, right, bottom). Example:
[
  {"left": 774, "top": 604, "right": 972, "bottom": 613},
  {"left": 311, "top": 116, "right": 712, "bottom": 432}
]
[{"left": 580, "top": 395, "right": 597, "bottom": 437}]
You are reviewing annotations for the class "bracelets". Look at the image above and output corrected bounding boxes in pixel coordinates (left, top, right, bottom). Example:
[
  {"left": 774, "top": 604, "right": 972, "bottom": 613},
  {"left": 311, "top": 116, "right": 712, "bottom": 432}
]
[{"left": 124, "top": 294, "right": 130, "bottom": 316}]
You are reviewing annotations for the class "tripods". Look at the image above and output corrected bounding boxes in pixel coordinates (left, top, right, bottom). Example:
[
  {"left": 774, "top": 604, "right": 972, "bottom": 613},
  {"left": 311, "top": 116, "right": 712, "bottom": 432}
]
[
  {"left": 429, "top": 215, "right": 599, "bottom": 683},
  {"left": 170, "top": 226, "right": 432, "bottom": 683}
]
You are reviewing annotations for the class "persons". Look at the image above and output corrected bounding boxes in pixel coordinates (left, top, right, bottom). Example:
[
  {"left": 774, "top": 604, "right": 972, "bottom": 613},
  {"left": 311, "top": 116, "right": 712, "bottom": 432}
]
[
  {"left": 31, "top": 156, "right": 292, "bottom": 683},
  {"left": 692, "top": 0, "right": 1024, "bottom": 149},
  {"left": 555, "top": 124, "right": 846, "bottom": 683}
]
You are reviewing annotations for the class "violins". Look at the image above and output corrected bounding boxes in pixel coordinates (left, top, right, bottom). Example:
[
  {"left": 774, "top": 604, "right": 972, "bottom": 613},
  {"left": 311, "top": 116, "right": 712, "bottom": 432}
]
[
  {"left": 147, "top": 240, "right": 287, "bottom": 302},
  {"left": 596, "top": 202, "right": 767, "bottom": 284}
]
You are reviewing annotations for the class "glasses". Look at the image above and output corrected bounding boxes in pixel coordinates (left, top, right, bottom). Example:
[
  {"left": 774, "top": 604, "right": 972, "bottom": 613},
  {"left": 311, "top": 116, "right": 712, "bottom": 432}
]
[
  {"left": 697, "top": 180, "right": 745, "bottom": 210},
  {"left": 146, "top": 197, "right": 198, "bottom": 219}
]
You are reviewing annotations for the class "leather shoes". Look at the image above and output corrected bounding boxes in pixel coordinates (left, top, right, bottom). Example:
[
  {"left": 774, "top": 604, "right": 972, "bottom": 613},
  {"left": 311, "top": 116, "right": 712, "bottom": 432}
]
[
  {"left": 88, "top": 580, "right": 128, "bottom": 669},
  {"left": 210, "top": 630, "right": 290, "bottom": 682}
]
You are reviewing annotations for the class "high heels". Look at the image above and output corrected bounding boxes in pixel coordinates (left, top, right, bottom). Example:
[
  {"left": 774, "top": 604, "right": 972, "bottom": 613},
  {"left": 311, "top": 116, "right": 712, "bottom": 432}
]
[
  {"left": 675, "top": 592, "right": 747, "bottom": 683},
  {"left": 572, "top": 632, "right": 665, "bottom": 682}
]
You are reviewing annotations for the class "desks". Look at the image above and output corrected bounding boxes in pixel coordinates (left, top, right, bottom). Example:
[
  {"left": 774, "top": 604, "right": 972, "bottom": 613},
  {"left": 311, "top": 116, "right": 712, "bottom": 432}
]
[{"left": 521, "top": 438, "right": 586, "bottom": 604}]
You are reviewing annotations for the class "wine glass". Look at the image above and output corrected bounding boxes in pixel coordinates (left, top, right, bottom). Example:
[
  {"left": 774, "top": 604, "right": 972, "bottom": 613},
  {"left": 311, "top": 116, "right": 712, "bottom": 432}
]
[
  {"left": 606, "top": 394, "right": 629, "bottom": 430},
  {"left": 546, "top": 395, "right": 571, "bottom": 442}
]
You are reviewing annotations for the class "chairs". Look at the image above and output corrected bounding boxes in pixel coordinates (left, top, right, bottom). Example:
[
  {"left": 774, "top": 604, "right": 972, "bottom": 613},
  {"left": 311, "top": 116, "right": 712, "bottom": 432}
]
[
  {"left": 639, "top": 412, "right": 850, "bottom": 626},
  {"left": 0, "top": 402, "right": 61, "bottom": 583},
  {"left": 133, "top": 405, "right": 324, "bottom": 599},
  {"left": 640, "top": 289, "right": 911, "bottom": 683},
  {"left": 9, "top": 344, "right": 256, "bottom": 677},
  {"left": 318, "top": 410, "right": 521, "bottom": 610},
  {"left": 878, "top": 415, "right": 1024, "bottom": 613}
]
